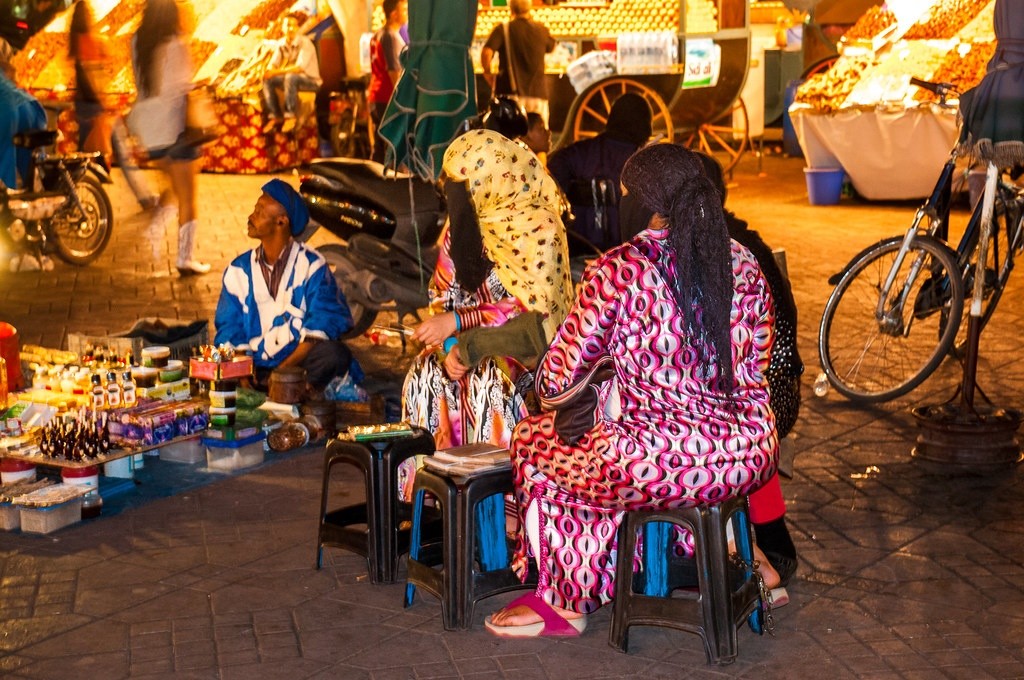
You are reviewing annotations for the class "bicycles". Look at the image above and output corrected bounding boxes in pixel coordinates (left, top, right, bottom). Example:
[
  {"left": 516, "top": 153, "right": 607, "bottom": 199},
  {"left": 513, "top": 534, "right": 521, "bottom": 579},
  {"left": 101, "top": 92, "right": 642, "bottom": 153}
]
[{"left": 818, "top": 78, "right": 1024, "bottom": 400}]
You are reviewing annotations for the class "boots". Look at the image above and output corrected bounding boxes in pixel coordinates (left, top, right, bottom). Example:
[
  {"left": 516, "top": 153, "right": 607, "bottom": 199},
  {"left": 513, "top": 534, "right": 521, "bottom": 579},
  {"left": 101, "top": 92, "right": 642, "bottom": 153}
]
[{"left": 175, "top": 219, "right": 210, "bottom": 273}]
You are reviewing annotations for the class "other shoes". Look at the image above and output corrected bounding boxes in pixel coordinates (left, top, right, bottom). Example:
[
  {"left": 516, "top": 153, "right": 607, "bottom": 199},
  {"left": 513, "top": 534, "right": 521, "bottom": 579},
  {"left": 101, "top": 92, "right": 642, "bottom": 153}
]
[
  {"left": 262, "top": 115, "right": 282, "bottom": 135},
  {"left": 282, "top": 113, "right": 306, "bottom": 135}
]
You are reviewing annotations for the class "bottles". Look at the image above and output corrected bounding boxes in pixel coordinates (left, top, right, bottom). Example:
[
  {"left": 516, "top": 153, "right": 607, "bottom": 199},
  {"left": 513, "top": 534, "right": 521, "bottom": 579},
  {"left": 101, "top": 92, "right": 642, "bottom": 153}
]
[{"left": 30, "top": 361, "right": 136, "bottom": 462}]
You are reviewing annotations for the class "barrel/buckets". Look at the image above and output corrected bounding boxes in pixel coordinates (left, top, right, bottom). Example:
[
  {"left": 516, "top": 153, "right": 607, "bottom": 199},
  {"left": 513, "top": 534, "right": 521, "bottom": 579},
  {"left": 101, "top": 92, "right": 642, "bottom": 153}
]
[
  {"left": 59, "top": 464, "right": 99, "bottom": 496},
  {"left": 0, "top": 458, "right": 37, "bottom": 486},
  {"left": 802, "top": 166, "right": 844, "bottom": 204},
  {"left": 104, "top": 452, "right": 146, "bottom": 478}
]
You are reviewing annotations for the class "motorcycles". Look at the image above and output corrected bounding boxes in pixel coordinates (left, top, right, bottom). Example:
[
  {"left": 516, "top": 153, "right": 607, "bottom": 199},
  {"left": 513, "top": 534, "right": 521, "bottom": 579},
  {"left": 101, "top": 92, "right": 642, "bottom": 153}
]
[
  {"left": 298, "top": 91, "right": 608, "bottom": 337},
  {"left": 0, "top": 130, "right": 117, "bottom": 272}
]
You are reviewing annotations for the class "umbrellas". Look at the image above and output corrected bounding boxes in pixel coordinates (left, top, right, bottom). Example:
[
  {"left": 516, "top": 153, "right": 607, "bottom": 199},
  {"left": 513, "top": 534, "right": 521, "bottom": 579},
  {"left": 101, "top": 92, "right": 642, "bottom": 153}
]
[
  {"left": 960, "top": 0, "right": 1024, "bottom": 414},
  {"left": 378, "top": 0, "right": 479, "bottom": 181}
]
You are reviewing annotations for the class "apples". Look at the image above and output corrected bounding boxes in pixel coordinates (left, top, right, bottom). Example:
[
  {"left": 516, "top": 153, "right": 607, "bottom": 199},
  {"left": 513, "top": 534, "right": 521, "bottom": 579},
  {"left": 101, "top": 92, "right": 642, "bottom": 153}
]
[{"left": 474, "top": 0, "right": 717, "bottom": 34}]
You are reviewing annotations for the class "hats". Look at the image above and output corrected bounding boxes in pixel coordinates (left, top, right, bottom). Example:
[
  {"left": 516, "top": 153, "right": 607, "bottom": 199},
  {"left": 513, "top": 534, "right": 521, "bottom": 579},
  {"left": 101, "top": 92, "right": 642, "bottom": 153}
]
[{"left": 261, "top": 178, "right": 309, "bottom": 238}]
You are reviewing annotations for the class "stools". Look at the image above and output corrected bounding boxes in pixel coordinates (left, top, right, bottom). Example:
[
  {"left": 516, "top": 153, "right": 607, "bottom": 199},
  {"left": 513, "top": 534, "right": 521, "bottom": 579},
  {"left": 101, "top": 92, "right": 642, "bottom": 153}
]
[
  {"left": 608, "top": 494, "right": 763, "bottom": 667},
  {"left": 312, "top": 424, "right": 445, "bottom": 588},
  {"left": 403, "top": 441, "right": 538, "bottom": 633}
]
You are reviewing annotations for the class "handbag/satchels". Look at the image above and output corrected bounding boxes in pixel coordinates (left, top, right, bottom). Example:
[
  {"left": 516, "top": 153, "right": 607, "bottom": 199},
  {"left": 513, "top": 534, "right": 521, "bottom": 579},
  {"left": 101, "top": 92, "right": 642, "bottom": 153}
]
[
  {"left": 164, "top": 81, "right": 221, "bottom": 159},
  {"left": 554, "top": 362, "right": 617, "bottom": 447}
]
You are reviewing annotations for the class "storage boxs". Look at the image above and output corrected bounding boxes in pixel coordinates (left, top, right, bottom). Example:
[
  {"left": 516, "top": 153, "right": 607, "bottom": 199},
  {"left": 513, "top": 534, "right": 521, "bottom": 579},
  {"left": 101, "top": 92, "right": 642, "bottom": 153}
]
[
  {"left": 0, "top": 494, "right": 85, "bottom": 538},
  {"left": 193, "top": 355, "right": 254, "bottom": 382},
  {"left": 98, "top": 473, "right": 136, "bottom": 518},
  {"left": 67, "top": 322, "right": 207, "bottom": 368},
  {"left": 157, "top": 423, "right": 267, "bottom": 471}
]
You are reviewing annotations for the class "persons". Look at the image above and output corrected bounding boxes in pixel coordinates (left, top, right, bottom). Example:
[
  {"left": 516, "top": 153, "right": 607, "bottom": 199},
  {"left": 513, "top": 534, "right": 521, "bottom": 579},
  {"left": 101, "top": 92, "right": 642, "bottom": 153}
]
[
  {"left": 68, "top": 0, "right": 170, "bottom": 210},
  {"left": 368, "top": 0, "right": 406, "bottom": 164},
  {"left": 261, "top": 17, "right": 321, "bottom": 132},
  {"left": 0, "top": 0, "right": 53, "bottom": 79},
  {"left": 697, "top": 150, "right": 801, "bottom": 589},
  {"left": 485, "top": 142, "right": 790, "bottom": 637},
  {"left": 481, "top": 0, "right": 555, "bottom": 112},
  {"left": 399, "top": 130, "right": 574, "bottom": 549},
  {"left": 213, "top": 179, "right": 354, "bottom": 405},
  {"left": 130, "top": 0, "right": 211, "bottom": 274}
]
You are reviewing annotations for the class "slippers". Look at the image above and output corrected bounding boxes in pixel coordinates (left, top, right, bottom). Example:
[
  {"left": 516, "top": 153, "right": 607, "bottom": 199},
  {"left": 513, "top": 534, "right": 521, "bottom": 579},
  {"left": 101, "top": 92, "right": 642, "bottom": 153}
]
[
  {"left": 483, "top": 590, "right": 588, "bottom": 640},
  {"left": 764, "top": 586, "right": 788, "bottom": 611}
]
